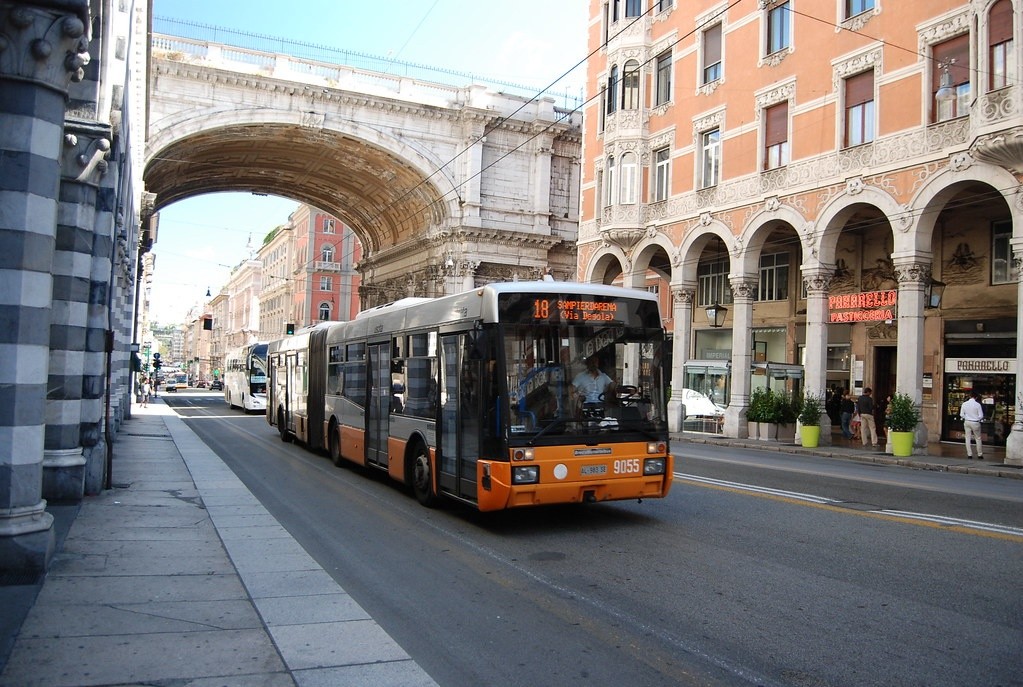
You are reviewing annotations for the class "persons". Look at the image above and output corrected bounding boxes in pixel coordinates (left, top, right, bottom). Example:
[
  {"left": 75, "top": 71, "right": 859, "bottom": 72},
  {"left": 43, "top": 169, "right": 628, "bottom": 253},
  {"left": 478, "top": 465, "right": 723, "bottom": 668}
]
[
  {"left": 993, "top": 391, "right": 1002, "bottom": 407},
  {"left": 256, "top": 385, "right": 266, "bottom": 393},
  {"left": 569, "top": 353, "right": 614, "bottom": 412},
  {"left": 141, "top": 377, "right": 151, "bottom": 408},
  {"left": 960, "top": 391, "right": 983, "bottom": 460},
  {"left": 136, "top": 376, "right": 161, "bottom": 392},
  {"left": 840, "top": 387, "right": 894, "bottom": 447}
]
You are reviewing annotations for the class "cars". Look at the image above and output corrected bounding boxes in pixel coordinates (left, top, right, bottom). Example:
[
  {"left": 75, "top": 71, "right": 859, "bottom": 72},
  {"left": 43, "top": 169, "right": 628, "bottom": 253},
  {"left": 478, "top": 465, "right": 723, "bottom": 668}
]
[
  {"left": 209, "top": 380, "right": 222, "bottom": 391},
  {"left": 197, "top": 381, "right": 205, "bottom": 388}
]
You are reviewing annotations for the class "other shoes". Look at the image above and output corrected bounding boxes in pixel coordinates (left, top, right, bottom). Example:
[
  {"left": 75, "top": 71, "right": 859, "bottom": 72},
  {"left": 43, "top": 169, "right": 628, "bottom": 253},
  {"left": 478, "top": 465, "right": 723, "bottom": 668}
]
[
  {"left": 873, "top": 443, "right": 880, "bottom": 447},
  {"left": 863, "top": 443, "right": 871, "bottom": 448},
  {"left": 968, "top": 456, "right": 972, "bottom": 459},
  {"left": 978, "top": 456, "right": 983, "bottom": 460},
  {"left": 849, "top": 435, "right": 853, "bottom": 440}
]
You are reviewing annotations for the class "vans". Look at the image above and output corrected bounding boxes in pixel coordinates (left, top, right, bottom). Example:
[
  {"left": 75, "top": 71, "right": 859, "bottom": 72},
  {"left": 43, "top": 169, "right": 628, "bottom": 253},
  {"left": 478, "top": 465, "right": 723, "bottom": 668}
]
[{"left": 166, "top": 379, "right": 178, "bottom": 392}]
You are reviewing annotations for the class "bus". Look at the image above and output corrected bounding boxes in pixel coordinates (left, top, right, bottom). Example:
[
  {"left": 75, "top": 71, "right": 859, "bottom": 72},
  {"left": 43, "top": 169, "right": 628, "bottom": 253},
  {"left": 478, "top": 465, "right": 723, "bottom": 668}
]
[
  {"left": 224, "top": 340, "right": 270, "bottom": 414},
  {"left": 170, "top": 371, "right": 189, "bottom": 388},
  {"left": 266, "top": 281, "right": 674, "bottom": 513}
]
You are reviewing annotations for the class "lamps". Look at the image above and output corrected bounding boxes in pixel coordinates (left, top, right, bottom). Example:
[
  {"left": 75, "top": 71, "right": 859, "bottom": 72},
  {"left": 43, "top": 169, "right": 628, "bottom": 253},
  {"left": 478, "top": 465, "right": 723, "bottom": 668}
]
[
  {"left": 704, "top": 236, "right": 729, "bottom": 327},
  {"left": 924, "top": 263, "right": 947, "bottom": 309}
]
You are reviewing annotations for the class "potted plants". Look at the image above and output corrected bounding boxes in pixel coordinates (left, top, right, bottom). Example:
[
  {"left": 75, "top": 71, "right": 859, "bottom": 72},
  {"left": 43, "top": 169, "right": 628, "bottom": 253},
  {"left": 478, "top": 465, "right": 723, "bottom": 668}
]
[
  {"left": 883, "top": 391, "right": 923, "bottom": 457},
  {"left": 744, "top": 386, "right": 795, "bottom": 443},
  {"left": 797, "top": 385, "right": 824, "bottom": 448}
]
